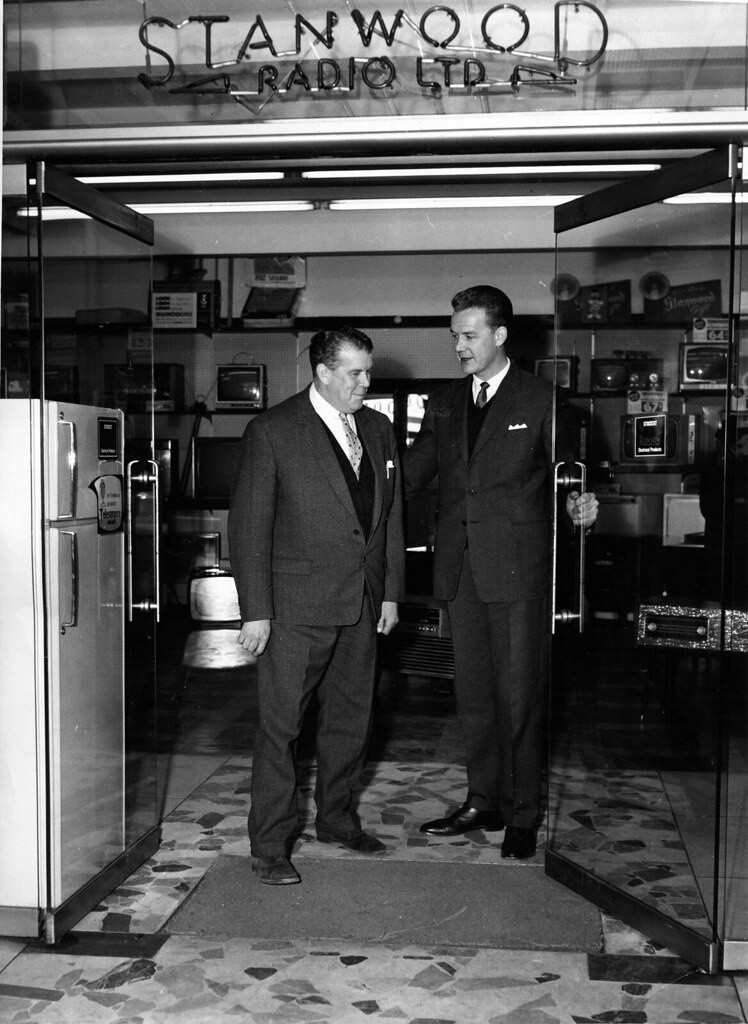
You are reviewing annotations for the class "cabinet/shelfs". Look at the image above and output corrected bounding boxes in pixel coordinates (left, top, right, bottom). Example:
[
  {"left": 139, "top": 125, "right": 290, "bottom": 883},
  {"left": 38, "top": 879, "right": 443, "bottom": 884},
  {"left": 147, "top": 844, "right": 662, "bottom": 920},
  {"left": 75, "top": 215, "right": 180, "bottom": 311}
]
[{"left": 31, "top": 306, "right": 741, "bottom": 724}]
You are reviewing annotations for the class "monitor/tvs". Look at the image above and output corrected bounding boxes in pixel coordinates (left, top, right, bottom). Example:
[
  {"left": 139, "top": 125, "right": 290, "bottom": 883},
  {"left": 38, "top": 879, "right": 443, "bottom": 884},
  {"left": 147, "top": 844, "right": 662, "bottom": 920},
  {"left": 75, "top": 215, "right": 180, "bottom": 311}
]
[
  {"left": 192, "top": 436, "right": 246, "bottom": 508},
  {"left": 588, "top": 358, "right": 628, "bottom": 392},
  {"left": 213, "top": 363, "right": 265, "bottom": 410},
  {"left": 619, "top": 412, "right": 700, "bottom": 465},
  {"left": 535, "top": 355, "right": 577, "bottom": 388},
  {"left": 678, "top": 343, "right": 729, "bottom": 383}
]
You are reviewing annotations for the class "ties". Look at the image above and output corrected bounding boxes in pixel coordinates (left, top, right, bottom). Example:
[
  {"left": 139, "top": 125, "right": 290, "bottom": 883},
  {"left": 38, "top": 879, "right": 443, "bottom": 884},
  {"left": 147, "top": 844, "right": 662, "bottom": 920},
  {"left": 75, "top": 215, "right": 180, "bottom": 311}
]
[
  {"left": 339, "top": 411, "right": 364, "bottom": 481},
  {"left": 476, "top": 381, "right": 489, "bottom": 409}
]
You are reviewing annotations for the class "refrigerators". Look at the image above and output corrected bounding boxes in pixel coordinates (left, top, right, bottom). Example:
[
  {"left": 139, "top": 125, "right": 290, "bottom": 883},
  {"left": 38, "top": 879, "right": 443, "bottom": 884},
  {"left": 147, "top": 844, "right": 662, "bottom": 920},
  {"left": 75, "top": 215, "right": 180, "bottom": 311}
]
[{"left": 0, "top": 399, "right": 125, "bottom": 910}]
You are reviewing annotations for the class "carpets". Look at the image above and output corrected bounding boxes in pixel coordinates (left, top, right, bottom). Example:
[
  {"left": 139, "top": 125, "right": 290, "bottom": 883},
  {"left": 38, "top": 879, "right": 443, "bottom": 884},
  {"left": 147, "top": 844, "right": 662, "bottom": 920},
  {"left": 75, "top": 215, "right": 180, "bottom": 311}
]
[{"left": 155, "top": 851, "right": 605, "bottom": 959}]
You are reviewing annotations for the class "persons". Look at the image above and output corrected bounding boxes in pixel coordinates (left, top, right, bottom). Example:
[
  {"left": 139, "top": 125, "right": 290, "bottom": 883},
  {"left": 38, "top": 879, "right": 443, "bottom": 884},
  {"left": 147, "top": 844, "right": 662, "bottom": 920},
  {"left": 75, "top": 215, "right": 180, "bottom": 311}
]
[
  {"left": 227, "top": 324, "right": 405, "bottom": 884},
  {"left": 401, "top": 285, "right": 599, "bottom": 860}
]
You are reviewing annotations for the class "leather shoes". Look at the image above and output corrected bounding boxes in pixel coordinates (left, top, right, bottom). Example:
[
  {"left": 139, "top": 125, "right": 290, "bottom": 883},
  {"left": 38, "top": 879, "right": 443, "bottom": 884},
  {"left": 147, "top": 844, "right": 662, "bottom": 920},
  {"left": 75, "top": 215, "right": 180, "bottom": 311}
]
[
  {"left": 419, "top": 805, "right": 506, "bottom": 836},
  {"left": 251, "top": 855, "right": 299, "bottom": 884},
  {"left": 317, "top": 826, "right": 385, "bottom": 854},
  {"left": 500, "top": 823, "right": 538, "bottom": 859}
]
